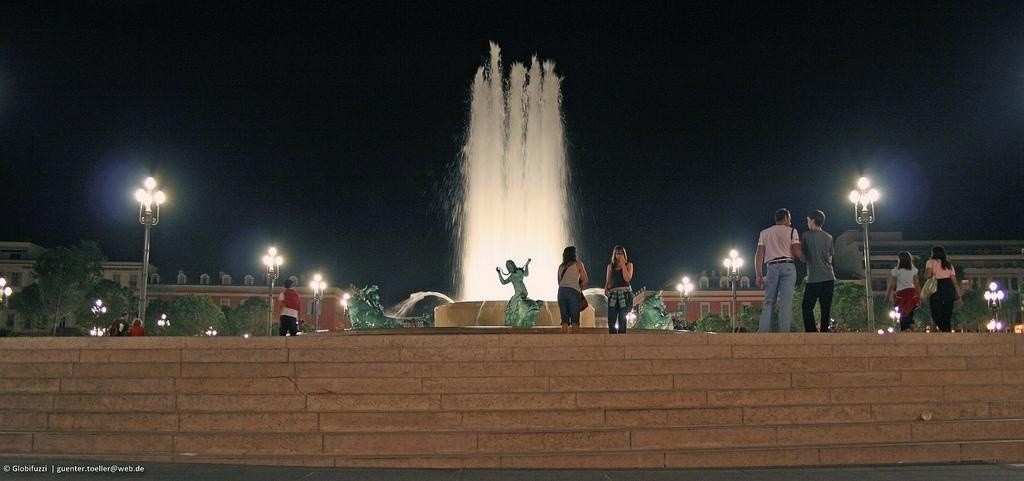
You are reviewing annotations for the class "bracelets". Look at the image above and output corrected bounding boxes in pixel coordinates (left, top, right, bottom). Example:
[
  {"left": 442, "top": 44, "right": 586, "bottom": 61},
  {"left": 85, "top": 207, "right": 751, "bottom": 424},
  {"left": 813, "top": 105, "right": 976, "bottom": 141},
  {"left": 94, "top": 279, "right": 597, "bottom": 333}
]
[{"left": 957, "top": 296, "right": 961, "bottom": 299}]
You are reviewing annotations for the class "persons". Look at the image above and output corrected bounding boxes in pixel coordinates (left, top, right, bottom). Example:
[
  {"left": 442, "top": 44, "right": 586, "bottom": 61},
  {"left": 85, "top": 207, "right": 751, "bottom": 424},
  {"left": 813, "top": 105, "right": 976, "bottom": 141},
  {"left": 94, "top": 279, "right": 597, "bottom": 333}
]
[
  {"left": 924, "top": 247, "right": 963, "bottom": 332},
  {"left": 802, "top": 210, "right": 837, "bottom": 333},
  {"left": 557, "top": 246, "right": 589, "bottom": 334},
  {"left": 497, "top": 258, "right": 531, "bottom": 325},
  {"left": 754, "top": 209, "right": 801, "bottom": 333},
  {"left": 110, "top": 313, "right": 130, "bottom": 337},
  {"left": 890, "top": 251, "right": 920, "bottom": 332},
  {"left": 604, "top": 245, "right": 634, "bottom": 333},
  {"left": 276, "top": 280, "right": 301, "bottom": 336},
  {"left": 129, "top": 320, "right": 144, "bottom": 336}
]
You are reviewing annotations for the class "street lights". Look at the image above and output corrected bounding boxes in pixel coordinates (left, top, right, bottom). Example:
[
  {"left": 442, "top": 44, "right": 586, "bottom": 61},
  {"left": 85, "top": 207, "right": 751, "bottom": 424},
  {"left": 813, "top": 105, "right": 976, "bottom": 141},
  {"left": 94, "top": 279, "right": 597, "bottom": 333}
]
[
  {"left": 627, "top": 311, "right": 636, "bottom": 330},
  {"left": 848, "top": 176, "right": 880, "bottom": 334},
  {"left": 134, "top": 176, "right": 167, "bottom": 329},
  {"left": 339, "top": 294, "right": 349, "bottom": 328},
  {"left": 987, "top": 319, "right": 1001, "bottom": 332},
  {"left": 90, "top": 297, "right": 106, "bottom": 337},
  {"left": 677, "top": 276, "right": 694, "bottom": 330},
  {"left": 0, "top": 277, "right": 12, "bottom": 307},
  {"left": 890, "top": 307, "right": 900, "bottom": 332},
  {"left": 984, "top": 281, "right": 1004, "bottom": 332},
  {"left": 309, "top": 273, "right": 328, "bottom": 331},
  {"left": 261, "top": 246, "right": 283, "bottom": 337},
  {"left": 722, "top": 249, "right": 745, "bottom": 334}
]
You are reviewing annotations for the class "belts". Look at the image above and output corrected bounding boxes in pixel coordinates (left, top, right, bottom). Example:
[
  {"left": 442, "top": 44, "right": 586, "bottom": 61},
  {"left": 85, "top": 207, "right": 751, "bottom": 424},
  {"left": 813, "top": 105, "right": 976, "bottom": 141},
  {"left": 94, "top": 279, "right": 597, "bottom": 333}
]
[{"left": 767, "top": 261, "right": 795, "bottom": 267}]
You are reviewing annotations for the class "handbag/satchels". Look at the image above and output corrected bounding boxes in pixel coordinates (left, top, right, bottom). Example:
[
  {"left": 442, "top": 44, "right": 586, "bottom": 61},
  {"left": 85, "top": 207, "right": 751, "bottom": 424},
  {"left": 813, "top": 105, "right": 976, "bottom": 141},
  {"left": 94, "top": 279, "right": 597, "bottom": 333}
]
[
  {"left": 921, "top": 277, "right": 938, "bottom": 298},
  {"left": 580, "top": 293, "right": 588, "bottom": 311}
]
